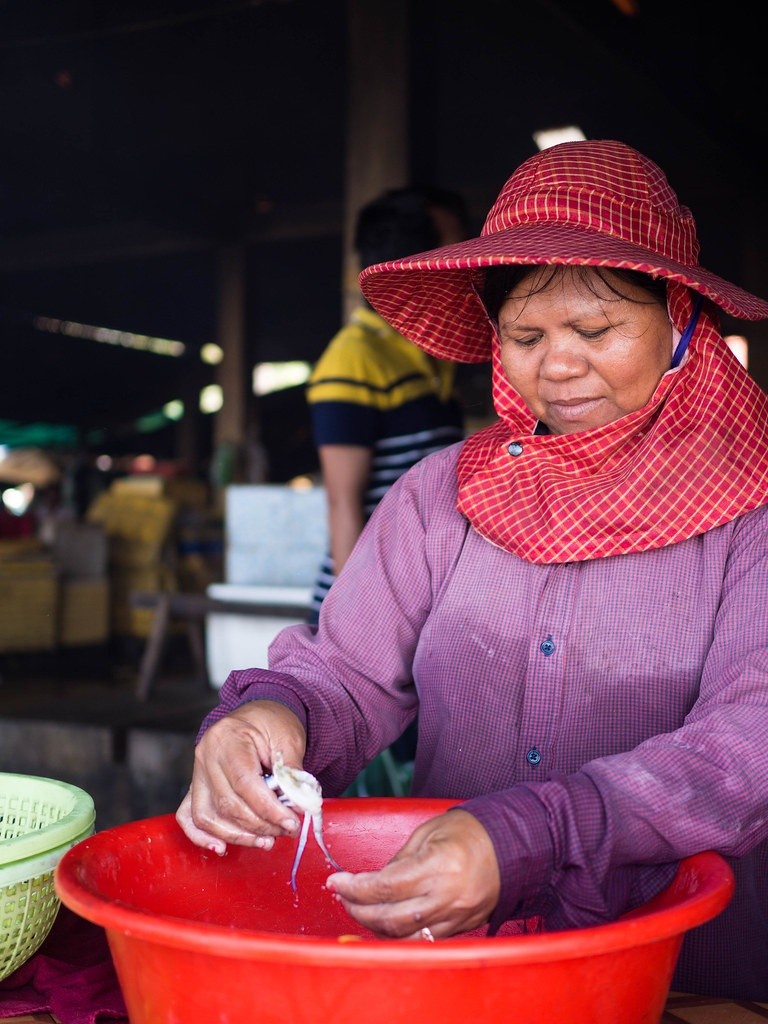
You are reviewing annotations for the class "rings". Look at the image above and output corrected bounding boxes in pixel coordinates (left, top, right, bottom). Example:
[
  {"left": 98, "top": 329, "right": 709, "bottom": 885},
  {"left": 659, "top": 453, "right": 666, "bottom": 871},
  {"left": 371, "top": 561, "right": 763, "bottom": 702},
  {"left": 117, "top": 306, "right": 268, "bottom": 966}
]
[{"left": 421, "top": 927, "right": 434, "bottom": 943}]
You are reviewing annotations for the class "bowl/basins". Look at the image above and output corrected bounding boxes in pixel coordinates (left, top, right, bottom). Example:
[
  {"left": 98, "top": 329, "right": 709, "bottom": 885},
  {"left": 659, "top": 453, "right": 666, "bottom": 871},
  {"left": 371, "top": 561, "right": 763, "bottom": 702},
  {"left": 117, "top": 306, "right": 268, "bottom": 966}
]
[{"left": 52, "top": 796, "right": 736, "bottom": 1023}]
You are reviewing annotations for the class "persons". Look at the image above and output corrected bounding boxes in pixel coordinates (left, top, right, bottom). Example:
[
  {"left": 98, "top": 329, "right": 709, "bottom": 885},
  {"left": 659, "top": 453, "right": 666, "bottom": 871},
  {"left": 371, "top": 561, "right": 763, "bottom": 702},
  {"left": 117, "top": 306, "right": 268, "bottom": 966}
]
[
  {"left": 306, "top": 186, "right": 463, "bottom": 623},
  {"left": 175, "top": 139, "right": 768, "bottom": 996}
]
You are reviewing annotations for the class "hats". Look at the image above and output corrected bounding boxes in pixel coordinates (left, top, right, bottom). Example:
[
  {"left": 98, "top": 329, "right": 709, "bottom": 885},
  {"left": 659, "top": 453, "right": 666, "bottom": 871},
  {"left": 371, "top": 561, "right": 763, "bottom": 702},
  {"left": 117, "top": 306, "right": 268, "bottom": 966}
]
[{"left": 357, "top": 140, "right": 768, "bottom": 563}]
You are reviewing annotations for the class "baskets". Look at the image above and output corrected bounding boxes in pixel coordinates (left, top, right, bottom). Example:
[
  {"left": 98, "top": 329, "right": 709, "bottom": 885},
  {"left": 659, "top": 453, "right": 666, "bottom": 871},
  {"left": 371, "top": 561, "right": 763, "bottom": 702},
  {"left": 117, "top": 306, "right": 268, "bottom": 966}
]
[{"left": 0, "top": 767, "right": 98, "bottom": 982}]
